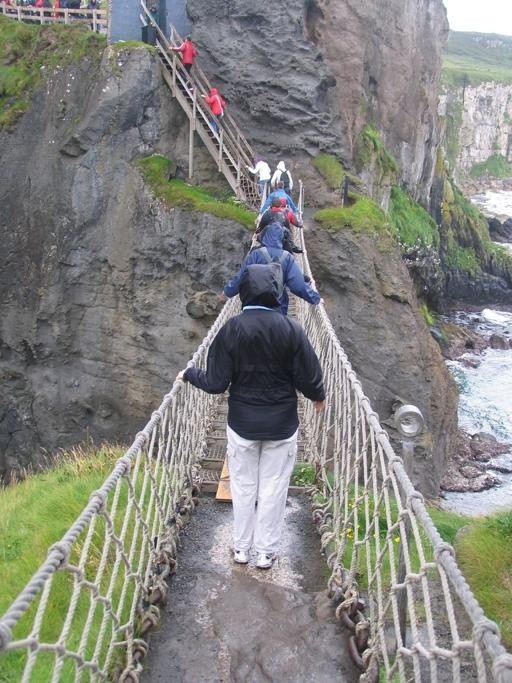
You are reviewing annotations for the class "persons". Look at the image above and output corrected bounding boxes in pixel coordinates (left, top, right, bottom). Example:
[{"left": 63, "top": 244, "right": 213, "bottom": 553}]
[
  {"left": 0, "top": 0, "right": 102, "bottom": 31},
  {"left": 145, "top": 7, "right": 161, "bottom": 48},
  {"left": 175, "top": 263, "right": 327, "bottom": 569},
  {"left": 245, "top": 154, "right": 271, "bottom": 196},
  {"left": 271, "top": 160, "right": 293, "bottom": 194},
  {"left": 201, "top": 88, "right": 225, "bottom": 143},
  {"left": 221, "top": 180, "right": 326, "bottom": 316},
  {"left": 168, "top": 36, "right": 197, "bottom": 90}
]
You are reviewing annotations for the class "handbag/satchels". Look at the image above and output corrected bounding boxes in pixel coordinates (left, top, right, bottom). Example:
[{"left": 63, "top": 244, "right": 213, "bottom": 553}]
[{"left": 218, "top": 108, "right": 223, "bottom": 118}]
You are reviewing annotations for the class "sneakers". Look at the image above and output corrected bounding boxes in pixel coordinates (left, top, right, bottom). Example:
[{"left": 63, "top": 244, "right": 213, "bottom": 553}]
[
  {"left": 257, "top": 552, "right": 276, "bottom": 568},
  {"left": 233, "top": 548, "right": 251, "bottom": 563}
]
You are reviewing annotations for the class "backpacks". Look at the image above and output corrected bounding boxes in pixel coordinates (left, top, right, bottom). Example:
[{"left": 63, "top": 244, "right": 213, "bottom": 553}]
[
  {"left": 258, "top": 247, "right": 289, "bottom": 299},
  {"left": 278, "top": 169, "right": 289, "bottom": 187}
]
